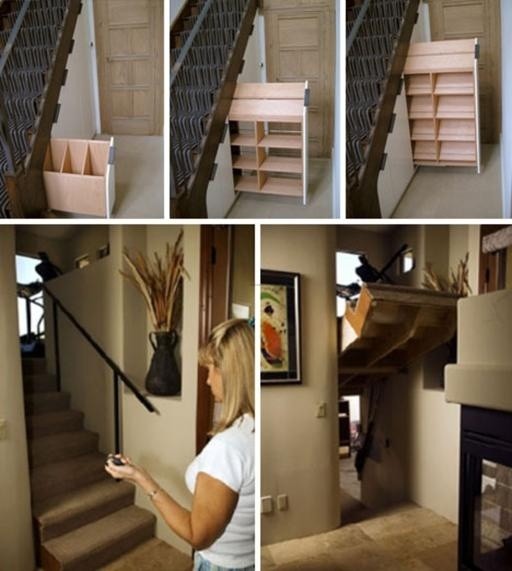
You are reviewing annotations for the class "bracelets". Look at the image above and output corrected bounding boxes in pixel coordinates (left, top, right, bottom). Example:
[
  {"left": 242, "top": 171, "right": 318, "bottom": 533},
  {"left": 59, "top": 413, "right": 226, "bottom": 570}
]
[{"left": 148, "top": 486, "right": 161, "bottom": 500}]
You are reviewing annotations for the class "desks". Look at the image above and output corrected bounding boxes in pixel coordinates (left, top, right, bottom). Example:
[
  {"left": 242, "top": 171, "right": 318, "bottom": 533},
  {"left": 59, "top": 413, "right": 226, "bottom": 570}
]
[{"left": 458, "top": 404, "right": 512, "bottom": 571}]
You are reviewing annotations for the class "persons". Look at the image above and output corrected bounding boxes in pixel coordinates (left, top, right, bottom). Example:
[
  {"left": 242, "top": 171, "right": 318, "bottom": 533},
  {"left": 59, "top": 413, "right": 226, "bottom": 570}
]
[{"left": 104, "top": 317, "right": 254, "bottom": 571}]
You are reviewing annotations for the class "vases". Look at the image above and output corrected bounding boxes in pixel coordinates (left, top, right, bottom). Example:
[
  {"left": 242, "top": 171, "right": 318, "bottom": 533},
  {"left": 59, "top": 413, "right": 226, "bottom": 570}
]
[{"left": 145, "top": 330, "right": 181, "bottom": 396}]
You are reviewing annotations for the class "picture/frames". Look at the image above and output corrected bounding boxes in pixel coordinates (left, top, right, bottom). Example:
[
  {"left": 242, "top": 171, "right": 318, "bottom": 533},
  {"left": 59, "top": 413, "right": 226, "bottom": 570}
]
[{"left": 261, "top": 269, "right": 302, "bottom": 385}]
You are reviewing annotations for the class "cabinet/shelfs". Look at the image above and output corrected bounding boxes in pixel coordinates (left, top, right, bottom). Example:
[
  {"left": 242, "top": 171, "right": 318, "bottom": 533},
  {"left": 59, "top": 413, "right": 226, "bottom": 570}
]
[
  {"left": 338, "top": 401, "right": 350, "bottom": 459},
  {"left": 403, "top": 39, "right": 481, "bottom": 173},
  {"left": 39, "top": 135, "right": 116, "bottom": 219},
  {"left": 229, "top": 81, "right": 310, "bottom": 205}
]
